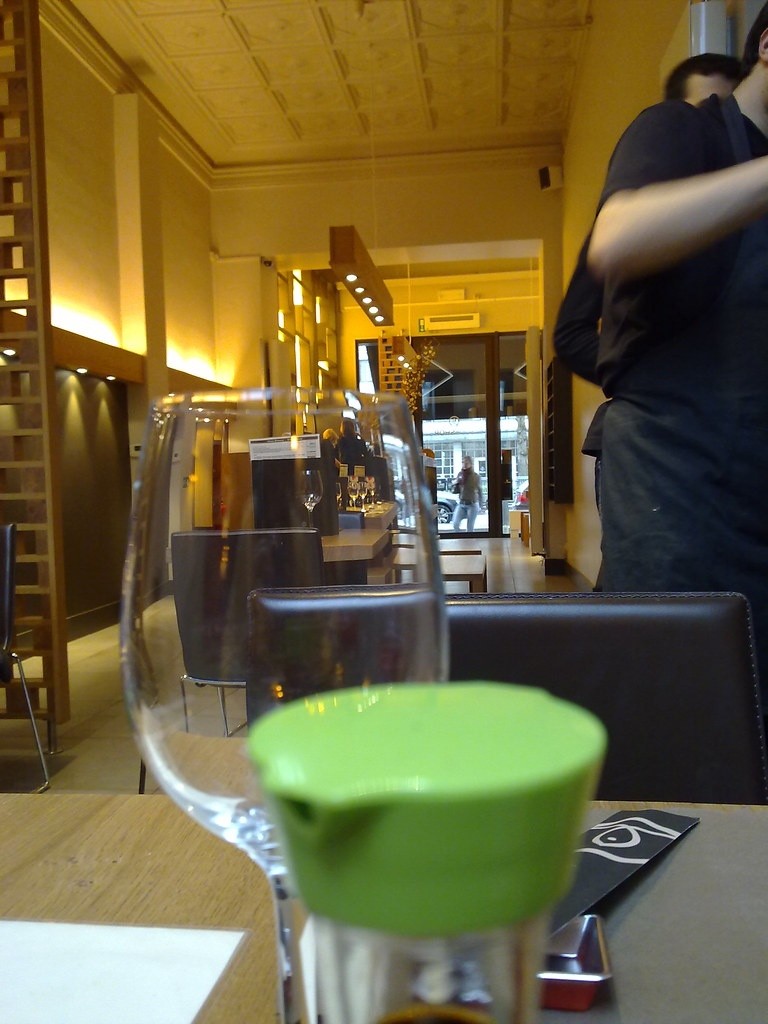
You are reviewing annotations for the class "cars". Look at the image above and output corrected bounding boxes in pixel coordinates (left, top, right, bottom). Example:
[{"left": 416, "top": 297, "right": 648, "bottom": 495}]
[
  {"left": 394, "top": 484, "right": 460, "bottom": 525},
  {"left": 511, "top": 481, "right": 529, "bottom": 511}
]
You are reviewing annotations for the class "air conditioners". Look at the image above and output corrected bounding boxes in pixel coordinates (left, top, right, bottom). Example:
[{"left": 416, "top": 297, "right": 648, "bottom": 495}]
[{"left": 424, "top": 312, "right": 480, "bottom": 331}]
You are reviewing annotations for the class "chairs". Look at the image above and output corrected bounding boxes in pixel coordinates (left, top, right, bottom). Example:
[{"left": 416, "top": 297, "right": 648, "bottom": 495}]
[
  {"left": 445, "top": 591, "right": 768, "bottom": 806},
  {"left": 169, "top": 528, "right": 328, "bottom": 737},
  {"left": 337, "top": 510, "right": 368, "bottom": 585},
  {"left": 0, "top": 523, "right": 52, "bottom": 794}
]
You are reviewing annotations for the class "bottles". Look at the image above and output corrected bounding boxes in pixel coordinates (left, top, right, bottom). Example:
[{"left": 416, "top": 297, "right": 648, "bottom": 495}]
[{"left": 249, "top": 680, "right": 606, "bottom": 1024}]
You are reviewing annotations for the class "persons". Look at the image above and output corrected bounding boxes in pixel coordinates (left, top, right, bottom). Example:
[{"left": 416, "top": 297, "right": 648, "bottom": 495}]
[
  {"left": 452, "top": 455, "right": 484, "bottom": 531},
  {"left": 553, "top": 0, "right": 768, "bottom": 666},
  {"left": 322, "top": 419, "right": 374, "bottom": 475}
]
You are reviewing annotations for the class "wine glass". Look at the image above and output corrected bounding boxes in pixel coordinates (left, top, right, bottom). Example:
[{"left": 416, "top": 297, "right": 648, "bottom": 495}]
[
  {"left": 368, "top": 482, "right": 375, "bottom": 509},
  {"left": 336, "top": 483, "right": 342, "bottom": 502},
  {"left": 301, "top": 470, "right": 323, "bottom": 527},
  {"left": 359, "top": 482, "right": 367, "bottom": 512},
  {"left": 122, "top": 387, "right": 448, "bottom": 1024},
  {"left": 348, "top": 482, "right": 359, "bottom": 510}
]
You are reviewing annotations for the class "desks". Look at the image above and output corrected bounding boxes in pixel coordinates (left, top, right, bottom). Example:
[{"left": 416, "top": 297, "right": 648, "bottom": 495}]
[
  {"left": 440, "top": 554, "right": 486, "bottom": 592},
  {"left": 0, "top": 793, "right": 768, "bottom": 1024},
  {"left": 224, "top": 527, "right": 389, "bottom": 586},
  {"left": 346, "top": 500, "right": 398, "bottom": 584}
]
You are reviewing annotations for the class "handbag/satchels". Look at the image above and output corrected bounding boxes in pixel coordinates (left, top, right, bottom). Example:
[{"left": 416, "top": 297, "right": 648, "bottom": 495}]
[{"left": 451, "top": 472, "right": 460, "bottom": 494}]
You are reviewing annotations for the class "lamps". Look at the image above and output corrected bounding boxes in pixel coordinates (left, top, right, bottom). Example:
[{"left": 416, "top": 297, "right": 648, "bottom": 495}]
[
  {"left": 392, "top": 256, "right": 418, "bottom": 372},
  {"left": 328, "top": 94, "right": 395, "bottom": 327}
]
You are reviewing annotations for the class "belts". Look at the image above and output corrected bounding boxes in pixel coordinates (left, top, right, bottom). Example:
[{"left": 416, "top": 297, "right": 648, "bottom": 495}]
[{"left": 460, "top": 501, "right": 474, "bottom": 505}]
